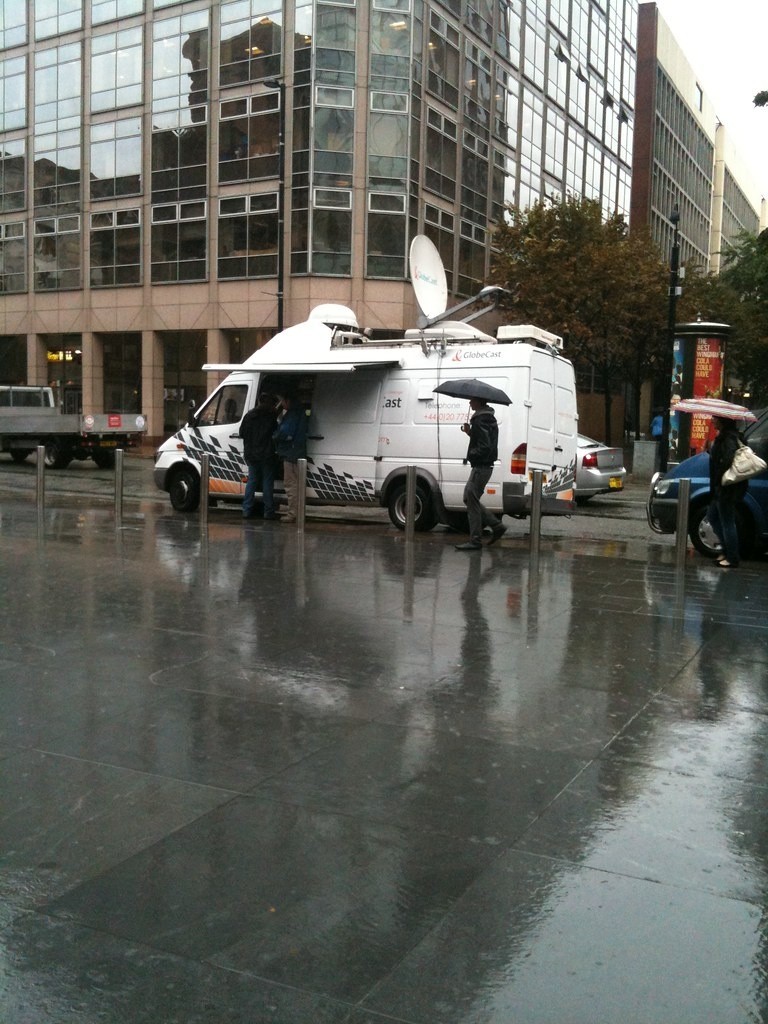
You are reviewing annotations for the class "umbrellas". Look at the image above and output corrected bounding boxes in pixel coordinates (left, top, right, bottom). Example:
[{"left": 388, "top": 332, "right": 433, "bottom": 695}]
[
  {"left": 432, "top": 378, "right": 513, "bottom": 432},
  {"left": 671, "top": 398, "right": 758, "bottom": 438}
]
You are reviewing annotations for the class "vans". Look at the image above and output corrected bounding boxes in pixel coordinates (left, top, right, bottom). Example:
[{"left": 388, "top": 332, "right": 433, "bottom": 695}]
[
  {"left": 152, "top": 234, "right": 580, "bottom": 531},
  {"left": 644, "top": 401, "right": 768, "bottom": 559}
]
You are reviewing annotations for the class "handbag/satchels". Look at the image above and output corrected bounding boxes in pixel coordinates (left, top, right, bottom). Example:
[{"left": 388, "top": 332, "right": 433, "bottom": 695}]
[{"left": 721, "top": 437, "right": 768, "bottom": 487}]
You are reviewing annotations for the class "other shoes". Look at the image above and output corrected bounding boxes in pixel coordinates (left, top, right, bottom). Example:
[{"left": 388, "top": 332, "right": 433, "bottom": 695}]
[
  {"left": 486, "top": 523, "right": 507, "bottom": 545},
  {"left": 455, "top": 541, "right": 482, "bottom": 550},
  {"left": 280, "top": 515, "right": 297, "bottom": 523},
  {"left": 244, "top": 512, "right": 279, "bottom": 522},
  {"left": 713, "top": 553, "right": 739, "bottom": 568}
]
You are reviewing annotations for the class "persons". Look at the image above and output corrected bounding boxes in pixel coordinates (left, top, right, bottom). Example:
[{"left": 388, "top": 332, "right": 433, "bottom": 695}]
[
  {"left": 240, "top": 395, "right": 308, "bottom": 522},
  {"left": 455, "top": 400, "right": 507, "bottom": 549},
  {"left": 48, "top": 378, "right": 58, "bottom": 407},
  {"left": 702, "top": 415, "right": 748, "bottom": 567}
]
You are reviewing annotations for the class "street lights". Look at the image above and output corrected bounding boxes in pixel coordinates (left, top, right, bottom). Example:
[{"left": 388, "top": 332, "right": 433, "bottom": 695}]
[
  {"left": 660, "top": 204, "right": 682, "bottom": 476},
  {"left": 265, "top": 77, "right": 283, "bottom": 334}
]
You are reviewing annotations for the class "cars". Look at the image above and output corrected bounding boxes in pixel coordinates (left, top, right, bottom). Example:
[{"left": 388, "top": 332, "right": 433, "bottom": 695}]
[{"left": 574, "top": 435, "right": 627, "bottom": 503}]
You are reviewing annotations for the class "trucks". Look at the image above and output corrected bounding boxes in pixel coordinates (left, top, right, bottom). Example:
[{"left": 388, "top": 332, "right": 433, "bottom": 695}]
[{"left": 0, "top": 384, "right": 148, "bottom": 469}]
